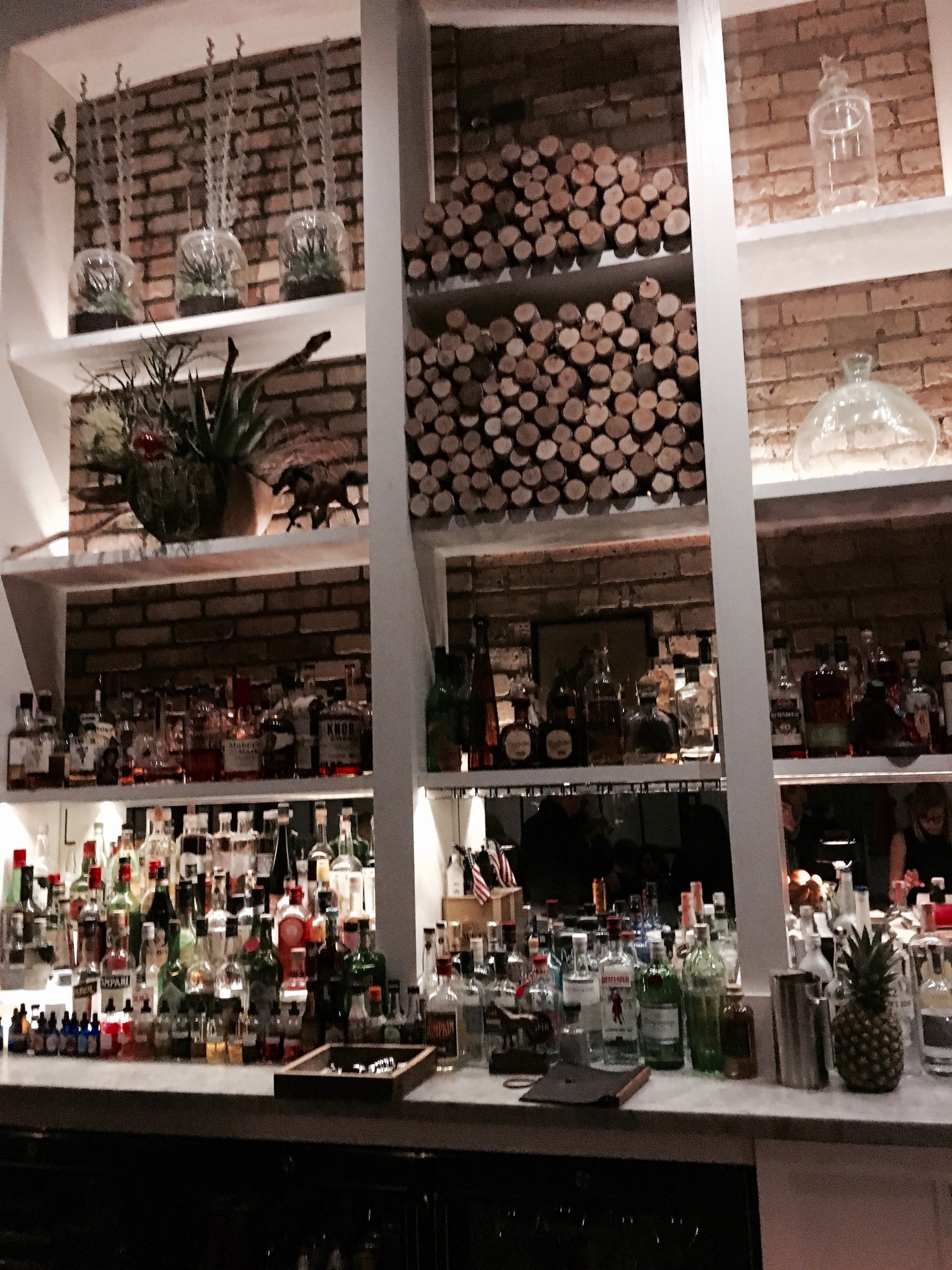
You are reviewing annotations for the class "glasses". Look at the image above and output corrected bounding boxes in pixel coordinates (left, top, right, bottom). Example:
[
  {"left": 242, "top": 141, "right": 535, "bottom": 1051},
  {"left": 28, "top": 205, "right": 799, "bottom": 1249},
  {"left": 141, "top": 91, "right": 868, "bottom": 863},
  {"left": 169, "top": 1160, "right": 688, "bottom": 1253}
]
[{"left": 921, "top": 814, "right": 946, "bottom": 823}]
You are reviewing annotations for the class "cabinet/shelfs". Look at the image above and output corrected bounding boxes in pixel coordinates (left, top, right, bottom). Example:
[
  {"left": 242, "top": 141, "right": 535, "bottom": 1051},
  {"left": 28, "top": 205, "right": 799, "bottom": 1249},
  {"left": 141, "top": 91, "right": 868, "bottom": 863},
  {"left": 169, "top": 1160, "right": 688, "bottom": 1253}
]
[{"left": 3, "top": 0, "right": 952, "bottom": 1267}]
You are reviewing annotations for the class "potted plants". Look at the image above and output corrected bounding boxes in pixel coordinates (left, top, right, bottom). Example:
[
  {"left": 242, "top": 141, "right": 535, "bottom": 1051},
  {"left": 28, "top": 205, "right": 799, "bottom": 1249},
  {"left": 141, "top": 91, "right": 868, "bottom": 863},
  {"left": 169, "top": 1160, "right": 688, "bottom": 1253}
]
[
  {"left": 276, "top": 208, "right": 347, "bottom": 303},
  {"left": 67, "top": 245, "right": 141, "bottom": 336},
  {"left": 174, "top": 226, "right": 251, "bottom": 318}
]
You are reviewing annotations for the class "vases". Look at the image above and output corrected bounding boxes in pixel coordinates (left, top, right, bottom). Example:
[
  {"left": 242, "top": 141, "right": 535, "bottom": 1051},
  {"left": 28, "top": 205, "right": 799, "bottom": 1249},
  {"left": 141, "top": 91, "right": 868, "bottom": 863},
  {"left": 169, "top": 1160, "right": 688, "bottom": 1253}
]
[{"left": 122, "top": 454, "right": 233, "bottom": 542}]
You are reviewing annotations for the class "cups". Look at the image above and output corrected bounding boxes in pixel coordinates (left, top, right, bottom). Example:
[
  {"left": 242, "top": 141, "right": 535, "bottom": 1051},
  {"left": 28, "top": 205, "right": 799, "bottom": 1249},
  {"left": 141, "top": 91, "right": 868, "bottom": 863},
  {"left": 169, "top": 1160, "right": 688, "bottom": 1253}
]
[{"left": 770, "top": 968, "right": 835, "bottom": 1089}]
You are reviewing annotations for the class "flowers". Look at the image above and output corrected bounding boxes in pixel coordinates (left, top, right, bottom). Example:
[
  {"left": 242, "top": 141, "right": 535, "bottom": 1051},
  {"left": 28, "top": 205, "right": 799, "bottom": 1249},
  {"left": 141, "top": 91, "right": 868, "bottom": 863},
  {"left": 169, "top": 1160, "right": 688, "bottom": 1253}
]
[{"left": 59, "top": 320, "right": 333, "bottom": 508}]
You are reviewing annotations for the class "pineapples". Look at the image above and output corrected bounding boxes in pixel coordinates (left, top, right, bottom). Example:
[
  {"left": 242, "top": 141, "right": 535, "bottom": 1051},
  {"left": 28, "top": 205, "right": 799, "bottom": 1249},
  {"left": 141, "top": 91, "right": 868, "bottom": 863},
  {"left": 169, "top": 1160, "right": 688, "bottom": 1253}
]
[{"left": 830, "top": 924, "right": 904, "bottom": 1092}]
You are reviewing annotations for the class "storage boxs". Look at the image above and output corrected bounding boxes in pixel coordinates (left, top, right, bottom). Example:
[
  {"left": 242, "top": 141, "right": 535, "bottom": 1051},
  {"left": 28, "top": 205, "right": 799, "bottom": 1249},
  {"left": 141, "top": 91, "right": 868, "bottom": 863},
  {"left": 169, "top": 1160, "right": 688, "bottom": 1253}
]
[{"left": 272, "top": 1040, "right": 439, "bottom": 1106}]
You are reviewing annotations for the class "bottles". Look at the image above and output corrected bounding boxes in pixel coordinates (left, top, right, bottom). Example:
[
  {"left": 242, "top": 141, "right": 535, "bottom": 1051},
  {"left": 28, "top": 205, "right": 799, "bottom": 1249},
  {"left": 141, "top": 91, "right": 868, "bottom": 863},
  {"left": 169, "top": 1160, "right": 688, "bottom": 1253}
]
[
  {"left": 6, "top": 663, "right": 373, "bottom": 789},
  {"left": 407, "top": 852, "right": 759, "bottom": 1081},
  {"left": 426, "top": 617, "right": 721, "bottom": 771},
  {"left": 771, "top": 620, "right": 950, "bottom": 758},
  {"left": 0, "top": 800, "right": 405, "bottom": 1064},
  {"left": 788, "top": 864, "right": 951, "bottom": 1079},
  {"left": 791, "top": 352, "right": 936, "bottom": 480},
  {"left": 805, "top": 55, "right": 880, "bottom": 215}
]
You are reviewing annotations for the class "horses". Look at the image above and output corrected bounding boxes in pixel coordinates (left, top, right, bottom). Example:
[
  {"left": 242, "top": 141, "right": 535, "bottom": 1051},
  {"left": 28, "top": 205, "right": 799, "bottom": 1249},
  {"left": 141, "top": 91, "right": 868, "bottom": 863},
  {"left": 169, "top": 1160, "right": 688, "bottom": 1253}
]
[{"left": 273, "top": 466, "right": 368, "bottom": 532}]
